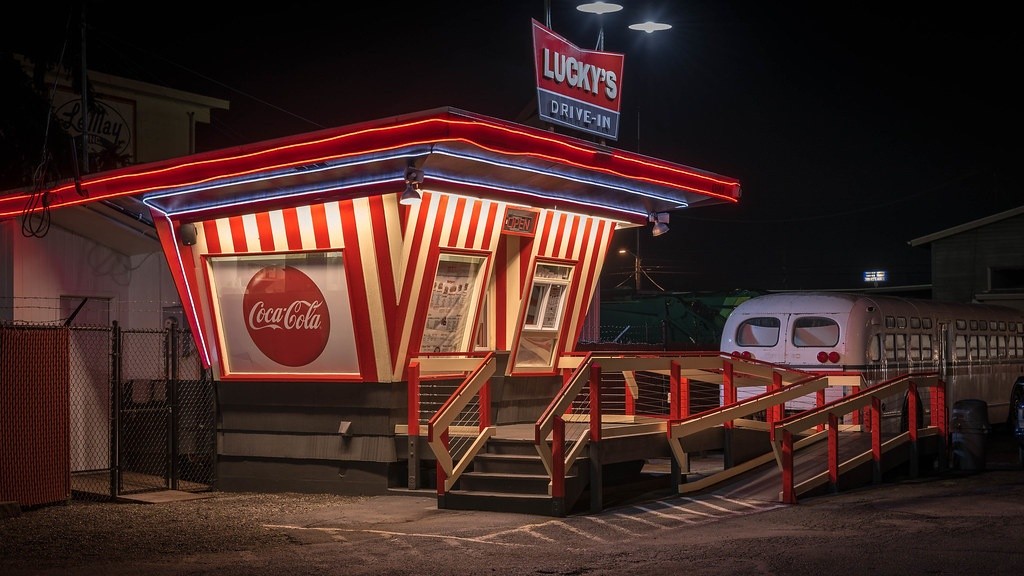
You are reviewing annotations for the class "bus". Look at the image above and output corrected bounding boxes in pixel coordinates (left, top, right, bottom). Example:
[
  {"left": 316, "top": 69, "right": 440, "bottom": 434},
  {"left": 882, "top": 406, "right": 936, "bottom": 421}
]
[{"left": 718, "top": 289, "right": 1024, "bottom": 446}]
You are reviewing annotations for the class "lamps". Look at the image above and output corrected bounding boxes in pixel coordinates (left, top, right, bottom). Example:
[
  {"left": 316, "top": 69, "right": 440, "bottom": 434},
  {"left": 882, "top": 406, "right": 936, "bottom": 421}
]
[
  {"left": 652, "top": 217, "right": 670, "bottom": 236},
  {"left": 399, "top": 184, "right": 422, "bottom": 205}
]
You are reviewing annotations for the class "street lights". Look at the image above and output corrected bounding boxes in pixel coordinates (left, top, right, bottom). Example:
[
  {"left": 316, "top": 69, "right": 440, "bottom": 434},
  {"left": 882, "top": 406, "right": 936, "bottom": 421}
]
[
  {"left": 628, "top": 7, "right": 675, "bottom": 290},
  {"left": 576, "top": 2, "right": 624, "bottom": 51}
]
